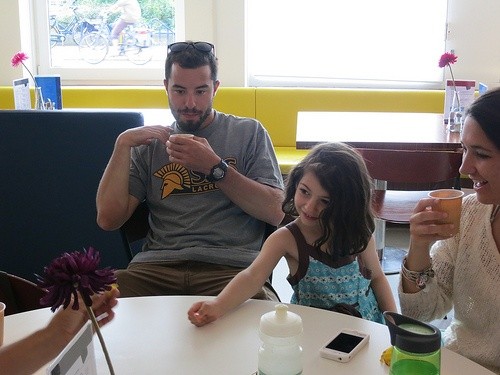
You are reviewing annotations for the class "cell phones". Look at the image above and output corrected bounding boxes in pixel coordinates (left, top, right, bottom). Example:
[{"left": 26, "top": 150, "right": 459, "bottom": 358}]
[{"left": 319, "top": 329, "right": 370, "bottom": 362}]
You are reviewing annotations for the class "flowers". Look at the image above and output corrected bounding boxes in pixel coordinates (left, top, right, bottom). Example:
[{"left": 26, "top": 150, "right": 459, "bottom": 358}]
[
  {"left": 11, "top": 51, "right": 38, "bottom": 87},
  {"left": 36, "top": 247, "right": 116, "bottom": 375},
  {"left": 439, "top": 52, "right": 460, "bottom": 110}
]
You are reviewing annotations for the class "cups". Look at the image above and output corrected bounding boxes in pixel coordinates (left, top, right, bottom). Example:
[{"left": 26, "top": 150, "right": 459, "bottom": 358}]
[
  {"left": 169, "top": 133, "right": 194, "bottom": 139},
  {"left": 0, "top": 302, "right": 6, "bottom": 345},
  {"left": 427, "top": 188, "right": 464, "bottom": 235}
]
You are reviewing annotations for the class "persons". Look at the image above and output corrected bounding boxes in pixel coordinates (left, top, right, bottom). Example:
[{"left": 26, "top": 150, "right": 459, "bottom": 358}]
[
  {"left": 398, "top": 86, "right": 500, "bottom": 375},
  {"left": 186, "top": 141, "right": 398, "bottom": 329},
  {"left": 0, "top": 245, "right": 120, "bottom": 375},
  {"left": 95, "top": 41, "right": 287, "bottom": 308}
]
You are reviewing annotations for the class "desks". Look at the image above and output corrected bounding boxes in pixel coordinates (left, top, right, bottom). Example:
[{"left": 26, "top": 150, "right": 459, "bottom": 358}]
[
  {"left": 4, "top": 296, "right": 497, "bottom": 375},
  {"left": 295, "top": 110, "right": 464, "bottom": 150}
]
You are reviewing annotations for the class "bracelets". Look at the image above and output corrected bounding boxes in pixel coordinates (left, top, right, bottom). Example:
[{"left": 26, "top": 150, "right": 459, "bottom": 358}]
[{"left": 401, "top": 253, "right": 434, "bottom": 287}]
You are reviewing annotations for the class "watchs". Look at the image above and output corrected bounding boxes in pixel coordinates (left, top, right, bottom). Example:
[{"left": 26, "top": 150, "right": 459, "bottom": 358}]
[{"left": 206, "top": 158, "right": 228, "bottom": 183}]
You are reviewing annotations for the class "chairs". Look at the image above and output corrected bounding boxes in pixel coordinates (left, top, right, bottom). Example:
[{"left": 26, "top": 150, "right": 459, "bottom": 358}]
[{"left": 355, "top": 147, "right": 465, "bottom": 263}]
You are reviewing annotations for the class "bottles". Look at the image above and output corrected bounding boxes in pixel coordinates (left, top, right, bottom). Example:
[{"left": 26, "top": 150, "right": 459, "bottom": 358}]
[
  {"left": 257, "top": 303, "right": 304, "bottom": 375},
  {"left": 382, "top": 310, "right": 442, "bottom": 375}
]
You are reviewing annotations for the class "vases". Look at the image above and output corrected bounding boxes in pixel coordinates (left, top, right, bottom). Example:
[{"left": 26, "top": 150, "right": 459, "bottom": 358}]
[
  {"left": 448, "top": 91, "right": 463, "bottom": 132},
  {"left": 33, "top": 87, "right": 46, "bottom": 111}
]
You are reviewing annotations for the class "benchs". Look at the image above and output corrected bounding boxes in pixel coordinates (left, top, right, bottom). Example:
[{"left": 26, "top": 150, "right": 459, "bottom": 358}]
[{"left": 0, "top": 87, "right": 447, "bottom": 174}]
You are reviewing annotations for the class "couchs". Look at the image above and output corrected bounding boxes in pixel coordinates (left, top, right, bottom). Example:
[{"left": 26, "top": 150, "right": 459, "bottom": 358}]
[{"left": 0, "top": 110, "right": 152, "bottom": 315}]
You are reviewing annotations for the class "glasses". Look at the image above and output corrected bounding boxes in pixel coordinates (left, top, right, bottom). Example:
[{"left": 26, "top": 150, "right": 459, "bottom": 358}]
[{"left": 167, "top": 42, "right": 215, "bottom": 56}]
